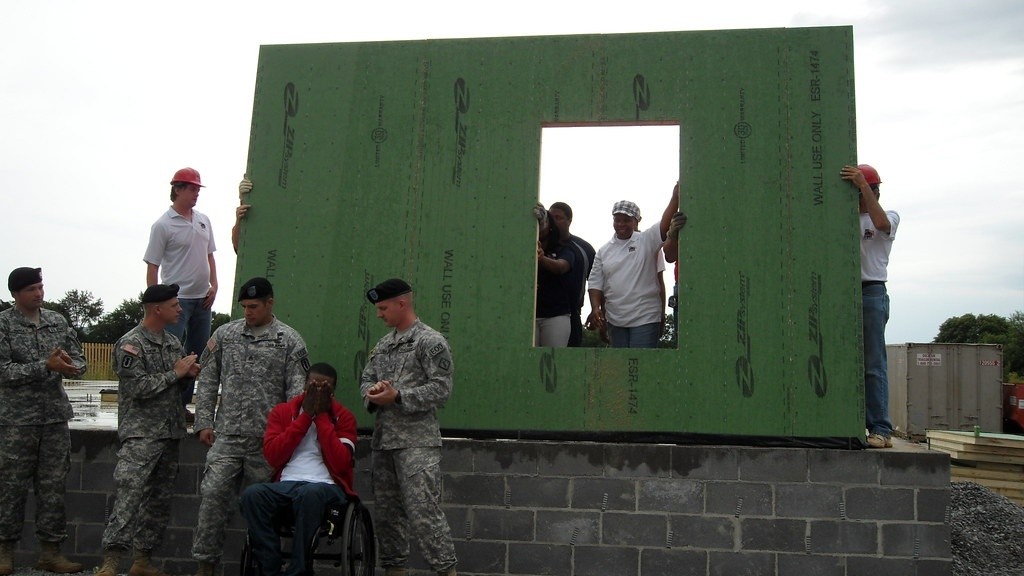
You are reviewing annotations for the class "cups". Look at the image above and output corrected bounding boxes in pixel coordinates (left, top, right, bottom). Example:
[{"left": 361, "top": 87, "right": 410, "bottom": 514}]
[{"left": 974, "top": 426, "right": 980, "bottom": 436}]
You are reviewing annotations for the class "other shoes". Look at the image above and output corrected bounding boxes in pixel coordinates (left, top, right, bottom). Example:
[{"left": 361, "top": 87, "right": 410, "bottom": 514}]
[
  {"left": 184, "top": 408, "right": 195, "bottom": 421},
  {"left": 284, "top": 563, "right": 315, "bottom": 576},
  {"left": 258, "top": 564, "right": 283, "bottom": 576}
]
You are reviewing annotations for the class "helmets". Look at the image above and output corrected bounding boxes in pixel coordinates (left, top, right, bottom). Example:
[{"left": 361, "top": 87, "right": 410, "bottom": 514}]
[
  {"left": 171, "top": 168, "right": 204, "bottom": 188},
  {"left": 858, "top": 165, "right": 882, "bottom": 185}
]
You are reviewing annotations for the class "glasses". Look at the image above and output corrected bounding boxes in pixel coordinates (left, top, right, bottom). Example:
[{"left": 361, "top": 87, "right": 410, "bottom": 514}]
[{"left": 871, "top": 186, "right": 879, "bottom": 191}]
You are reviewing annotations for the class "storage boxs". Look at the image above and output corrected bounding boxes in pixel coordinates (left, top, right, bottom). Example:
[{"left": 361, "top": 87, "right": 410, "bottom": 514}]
[{"left": 886, "top": 341, "right": 1004, "bottom": 440}]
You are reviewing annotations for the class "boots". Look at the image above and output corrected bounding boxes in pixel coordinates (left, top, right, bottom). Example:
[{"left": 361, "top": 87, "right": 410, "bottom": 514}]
[
  {"left": 386, "top": 567, "right": 408, "bottom": 576},
  {"left": 1, "top": 541, "right": 13, "bottom": 574},
  {"left": 193, "top": 561, "right": 212, "bottom": 576},
  {"left": 37, "top": 541, "right": 82, "bottom": 573},
  {"left": 130, "top": 549, "right": 167, "bottom": 576},
  {"left": 438, "top": 566, "right": 457, "bottom": 576},
  {"left": 94, "top": 547, "right": 120, "bottom": 576}
]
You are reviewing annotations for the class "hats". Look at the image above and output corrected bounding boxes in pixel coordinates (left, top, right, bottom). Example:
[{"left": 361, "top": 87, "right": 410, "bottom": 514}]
[
  {"left": 612, "top": 201, "right": 642, "bottom": 222},
  {"left": 238, "top": 277, "right": 273, "bottom": 302},
  {"left": 8, "top": 268, "right": 42, "bottom": 291},
  {"left": 143, "top": 283, "right": 179, "bottom": 303},
  {"left": 366, "top": 279, "right": 412, "bottom": 305}
]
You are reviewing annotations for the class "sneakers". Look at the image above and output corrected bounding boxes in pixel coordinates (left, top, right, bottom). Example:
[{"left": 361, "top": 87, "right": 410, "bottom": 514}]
[{"left": 867, "top": 433, "right": 893, "bottom": 447}]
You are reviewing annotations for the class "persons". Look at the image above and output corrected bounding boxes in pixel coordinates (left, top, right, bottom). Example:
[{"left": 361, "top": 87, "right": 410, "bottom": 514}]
[
  {"left": 663, "top": 212, "right": 687, "bottom": 338},
  {"left": 840, "top": 165, "right": 900, "bottom": 446},
  {"left": 588, "top": 181, "right": 679, "bottom": 348},
  {"left": 191, "top": 276, "right": 312, "bottom": 575},
  {"left": 239, "top": 362, "right": 358, "bottom": 576},
  {"left": 533, "top": 202, "right": 575, "bottom": 347},
  {"left": 94, "top": 284, "right": 201, "bottom": 576},
  {"left": 360, "top": 279, "right": 458, "bottom": 576},
  {"left": 549, "top": 202, "right": 596, "bottom": 347},
  {"left": 0, "top": 268, "right": 88, "bottom": 574},
  {"left": 232, "top": 178, "right": 252, "bottom": 254},
  {"left": 143, "top": 167, "right": 217, "bottom": 420}
]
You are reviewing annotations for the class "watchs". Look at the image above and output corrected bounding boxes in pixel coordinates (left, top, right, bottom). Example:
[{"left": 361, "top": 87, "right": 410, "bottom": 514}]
[{"left": 395, "top": 391, "right": 401, "bottom": 405}]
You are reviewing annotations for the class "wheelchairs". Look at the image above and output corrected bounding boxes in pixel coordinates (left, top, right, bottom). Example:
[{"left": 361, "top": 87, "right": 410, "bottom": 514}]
[{"left": 242, "top": 501, "right": 375, "bottom": 575}]
